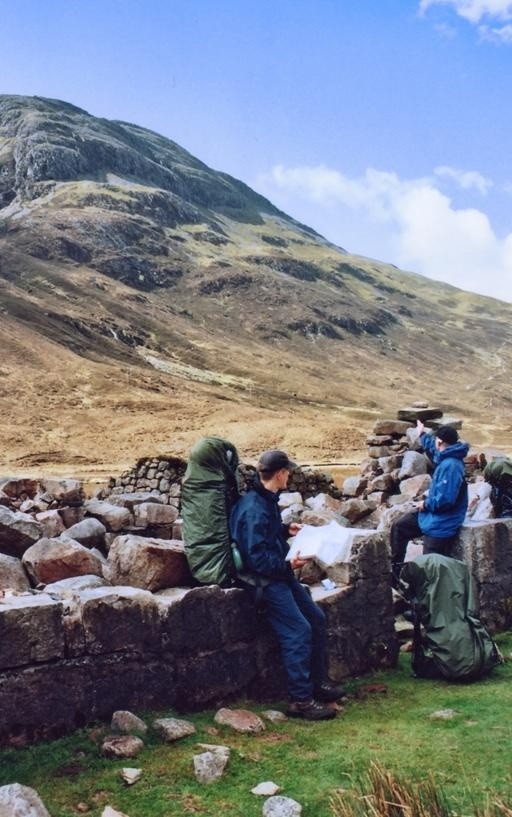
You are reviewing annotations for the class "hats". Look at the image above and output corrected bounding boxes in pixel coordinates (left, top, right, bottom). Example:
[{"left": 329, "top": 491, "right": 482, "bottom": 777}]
[
  {"left": 256, "top": 448, "right": 298, "bottom": 471},
  {"left": 431, "top": 424, "right": 460, "bottom": 445}
]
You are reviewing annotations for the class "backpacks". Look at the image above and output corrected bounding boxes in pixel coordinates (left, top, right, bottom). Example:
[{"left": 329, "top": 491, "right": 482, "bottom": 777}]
[
  {"left": 393, "top": 551, "right": 503, "bottom": 682},
  {"left": 176, "top": 433, "right": 241, "bottom": 587}
]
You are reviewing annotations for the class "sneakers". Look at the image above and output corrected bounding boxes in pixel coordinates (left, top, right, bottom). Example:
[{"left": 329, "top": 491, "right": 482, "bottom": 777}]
[
  {"left": 286, "top": 697, "right": 338, "bottom": 720},
  {"left": 314, "top": 678, "right": 346, "bottom": 704}
]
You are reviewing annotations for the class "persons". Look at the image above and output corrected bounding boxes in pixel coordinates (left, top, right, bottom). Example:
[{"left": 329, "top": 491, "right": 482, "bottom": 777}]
[
  {"left": 228, "top": 448, "right": 349, "bottom": 723},
  {"left": 387, "top": 418, "right": 471, "bottom": 623}
]
